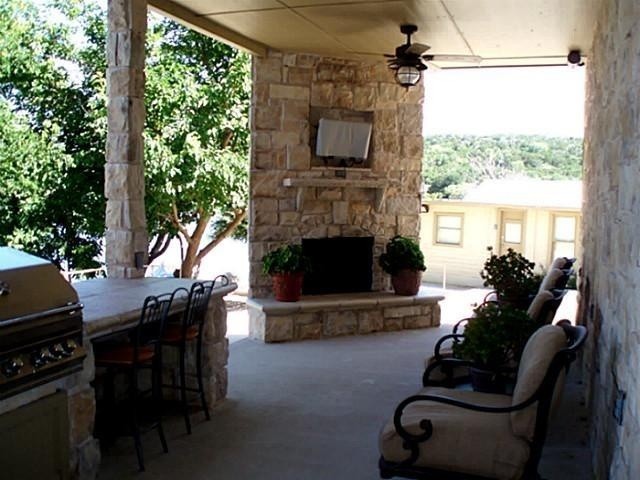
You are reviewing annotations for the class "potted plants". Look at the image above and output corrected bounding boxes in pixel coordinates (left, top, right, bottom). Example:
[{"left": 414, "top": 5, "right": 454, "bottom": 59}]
[
  {"left": 379, "top": 235, "right": 426, "bottom": 296},
  {"left": 261, "top": 245, "right": 313, "bottom": 302}
]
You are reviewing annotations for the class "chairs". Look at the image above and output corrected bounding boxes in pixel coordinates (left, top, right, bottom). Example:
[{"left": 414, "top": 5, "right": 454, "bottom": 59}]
[
  {"left": 96, "top": 276, "right": 228, "bottom": 472},
  {"left": 379, "top": 246, "right": 588, "bottom": 480}
]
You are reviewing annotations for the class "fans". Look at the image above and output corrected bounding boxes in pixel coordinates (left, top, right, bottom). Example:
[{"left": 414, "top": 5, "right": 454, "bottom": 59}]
[{"left": 346, "top": 24, "right": 482, "bottom": 70}]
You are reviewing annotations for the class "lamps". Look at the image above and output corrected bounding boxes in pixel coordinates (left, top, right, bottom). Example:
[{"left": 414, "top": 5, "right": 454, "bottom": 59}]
[{"left": 394, "top": 60, "right": 421, "bottom": 91}]
